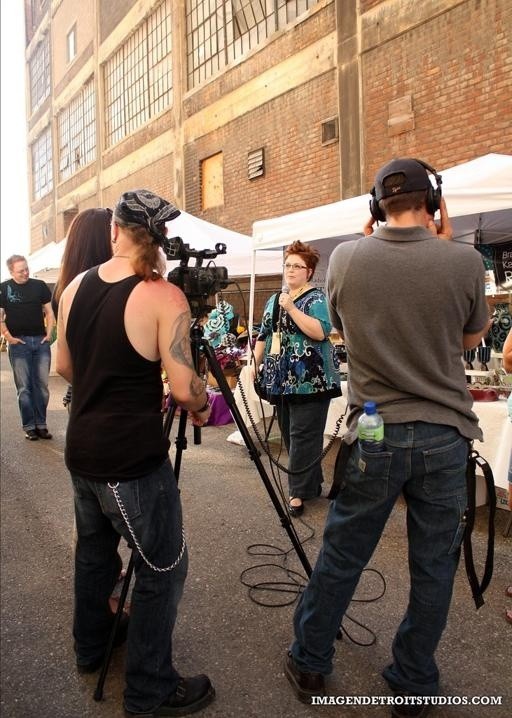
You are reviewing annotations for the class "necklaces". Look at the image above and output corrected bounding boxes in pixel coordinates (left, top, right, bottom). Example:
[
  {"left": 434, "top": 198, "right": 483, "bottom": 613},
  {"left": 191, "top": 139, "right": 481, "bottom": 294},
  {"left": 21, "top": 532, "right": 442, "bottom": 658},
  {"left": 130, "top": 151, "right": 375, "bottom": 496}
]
[
  {"left": 290, "top": 282, "right": 310, "bottom": 302},
  {"left": 112, "top": 256, "right": 136, "bottom": 260}
]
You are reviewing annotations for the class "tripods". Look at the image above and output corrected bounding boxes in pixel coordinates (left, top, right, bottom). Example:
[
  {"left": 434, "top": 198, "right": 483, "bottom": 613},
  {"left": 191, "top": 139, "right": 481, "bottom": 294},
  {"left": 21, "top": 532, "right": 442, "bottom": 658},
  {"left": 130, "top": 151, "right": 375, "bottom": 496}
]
[{"left": 93, "top": 324, "right": 344, "bottom": 698}]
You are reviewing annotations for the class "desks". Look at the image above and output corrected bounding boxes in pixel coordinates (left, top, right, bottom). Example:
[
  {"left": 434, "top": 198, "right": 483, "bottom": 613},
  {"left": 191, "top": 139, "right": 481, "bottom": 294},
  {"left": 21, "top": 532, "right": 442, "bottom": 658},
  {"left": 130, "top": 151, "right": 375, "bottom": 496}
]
[{"left": 225, "top": 362, "right": 512, "bottom": 538}]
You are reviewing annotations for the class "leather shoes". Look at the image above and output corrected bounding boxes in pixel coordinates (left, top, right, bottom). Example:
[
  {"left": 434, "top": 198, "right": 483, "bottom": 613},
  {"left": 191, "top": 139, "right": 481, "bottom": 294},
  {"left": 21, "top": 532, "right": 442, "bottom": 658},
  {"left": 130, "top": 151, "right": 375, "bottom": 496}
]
[
  {"left": 390, "top": 691, "right": 437, "bottom": 718},
  {"left": 281, "top": 649, "right": 330, "bottom": 707},
  {"left": 122, "top": 671, "right": 217, "bottom": 718},
  {"left": 37, "top": 428, "right": 54, "bottom": 440},
  {"left": 25, "top": 430, "right": 39, "bottom": 440},
  {"left": 318, "top": 483, "right": 323, "bottom": 497},
  {"left": 286, "top": 495, "right": 305, "bottom": 517},
  {"left": 72, "top": 604, "right": 130, "bottom": 677}
]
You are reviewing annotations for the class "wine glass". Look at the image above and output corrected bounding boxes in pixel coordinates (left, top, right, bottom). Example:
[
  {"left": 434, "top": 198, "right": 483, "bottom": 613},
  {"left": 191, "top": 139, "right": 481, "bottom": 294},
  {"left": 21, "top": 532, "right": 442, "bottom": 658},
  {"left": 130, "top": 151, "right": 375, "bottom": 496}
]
[
  {"left": 477, "top": 346, "right": 491, "bottom": 372},
  {"left": 463, "top": 345, "right": 475, "bottom": 371}
]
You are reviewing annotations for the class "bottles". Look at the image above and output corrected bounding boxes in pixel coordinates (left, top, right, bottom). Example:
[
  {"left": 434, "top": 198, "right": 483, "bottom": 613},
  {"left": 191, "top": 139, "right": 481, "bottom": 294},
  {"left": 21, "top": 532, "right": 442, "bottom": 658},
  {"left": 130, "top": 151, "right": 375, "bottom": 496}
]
[{"left": 357, "top": 401, "right": 387, "bottom": 453}]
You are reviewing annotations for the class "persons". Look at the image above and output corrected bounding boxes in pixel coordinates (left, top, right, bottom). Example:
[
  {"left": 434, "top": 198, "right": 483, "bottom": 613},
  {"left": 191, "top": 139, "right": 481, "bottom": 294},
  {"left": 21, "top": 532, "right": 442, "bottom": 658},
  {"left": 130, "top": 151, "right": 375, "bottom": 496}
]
[
  {"left": 0, "top": 256, "right": 53, "bottom": 440},
  {"left": 56, "top": 189, "right": 214, "bottom": 718},
  {"left": 251, "top": 241, "right": 342, "bottom": 516},
  {"left": 280, "top": 159, "right": 493, "bottom": 718},
  {"left": 48, "top": 209, "right": 131, "bottom": 615},
  {"left": 502, "top": 327, "right": 512, "bottom": 624}
]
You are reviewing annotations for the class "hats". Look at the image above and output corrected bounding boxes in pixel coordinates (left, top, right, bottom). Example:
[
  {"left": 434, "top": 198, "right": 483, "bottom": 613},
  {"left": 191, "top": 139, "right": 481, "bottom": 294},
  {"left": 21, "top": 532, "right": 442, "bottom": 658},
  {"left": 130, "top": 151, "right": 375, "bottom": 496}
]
[{"left": 372, "top": 156, "right": 432, "bottom": 202}]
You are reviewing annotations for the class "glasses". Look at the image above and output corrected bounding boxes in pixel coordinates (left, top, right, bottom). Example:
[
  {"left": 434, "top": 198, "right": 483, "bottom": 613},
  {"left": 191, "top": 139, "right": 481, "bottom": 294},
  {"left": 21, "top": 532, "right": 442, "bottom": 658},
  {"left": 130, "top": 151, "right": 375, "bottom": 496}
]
[{"left": 281, "top": 262, "right": 310, "bottom": 271}]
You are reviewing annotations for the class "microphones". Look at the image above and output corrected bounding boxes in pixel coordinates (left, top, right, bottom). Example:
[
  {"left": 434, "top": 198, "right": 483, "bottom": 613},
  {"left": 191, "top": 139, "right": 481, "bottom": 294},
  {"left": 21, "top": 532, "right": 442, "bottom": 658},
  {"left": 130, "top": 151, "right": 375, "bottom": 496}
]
[{"left": 277, "top": 285, "right": 290, "bottom": 326}]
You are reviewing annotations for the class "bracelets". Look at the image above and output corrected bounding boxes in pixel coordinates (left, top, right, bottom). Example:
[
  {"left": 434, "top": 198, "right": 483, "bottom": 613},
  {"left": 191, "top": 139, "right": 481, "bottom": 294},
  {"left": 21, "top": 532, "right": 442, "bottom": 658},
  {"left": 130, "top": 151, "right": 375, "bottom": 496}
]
[{"left": 198, "top": 393, "right": 212, "bottom": 413}]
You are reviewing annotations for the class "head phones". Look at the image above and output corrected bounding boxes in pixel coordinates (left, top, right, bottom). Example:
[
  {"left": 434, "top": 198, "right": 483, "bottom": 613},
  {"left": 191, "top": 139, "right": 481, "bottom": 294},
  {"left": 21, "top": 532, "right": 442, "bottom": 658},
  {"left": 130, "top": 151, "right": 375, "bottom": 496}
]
[{"left": 368, "top": 157, "right": 442, "bottom": 222}]
